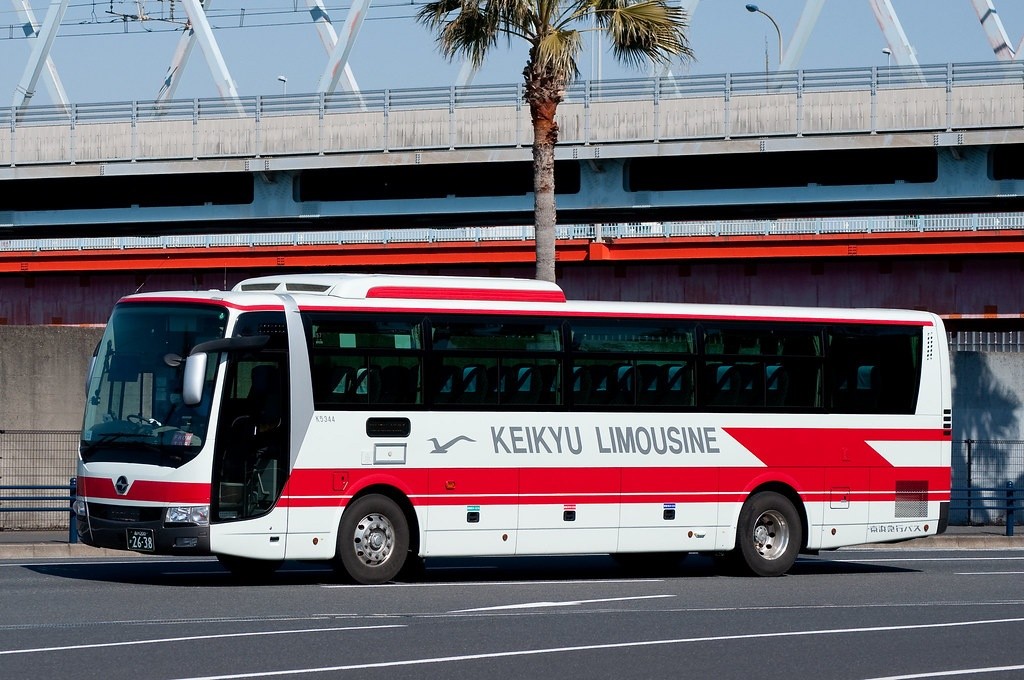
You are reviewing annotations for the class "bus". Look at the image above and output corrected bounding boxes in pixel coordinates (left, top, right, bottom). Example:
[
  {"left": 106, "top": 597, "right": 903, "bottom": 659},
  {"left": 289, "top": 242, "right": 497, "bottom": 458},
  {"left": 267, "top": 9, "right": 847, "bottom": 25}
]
[{"left": 69, "top": 271, "right": 953, "bottom": 585}]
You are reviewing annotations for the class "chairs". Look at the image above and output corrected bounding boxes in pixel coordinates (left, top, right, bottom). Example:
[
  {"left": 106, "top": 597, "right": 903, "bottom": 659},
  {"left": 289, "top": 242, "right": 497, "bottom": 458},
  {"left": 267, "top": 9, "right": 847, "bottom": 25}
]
[{"left": 195, "top": 363, "right": 896, "bottom": 442}]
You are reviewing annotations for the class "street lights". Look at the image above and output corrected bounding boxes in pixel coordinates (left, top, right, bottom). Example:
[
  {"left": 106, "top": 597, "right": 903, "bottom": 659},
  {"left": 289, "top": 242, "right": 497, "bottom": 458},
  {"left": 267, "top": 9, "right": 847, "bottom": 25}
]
[
  {"left": 746, "top": 3, "right": 782, "bottom": 70},
  {"left": 277, "top": 74, "right": 288, "bottom": 112},
  {"left": 881, "top": 48, "right": 892, "bottom": 86}
]
